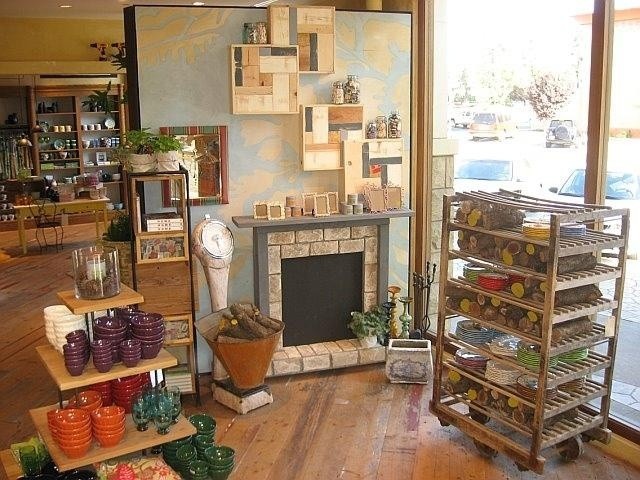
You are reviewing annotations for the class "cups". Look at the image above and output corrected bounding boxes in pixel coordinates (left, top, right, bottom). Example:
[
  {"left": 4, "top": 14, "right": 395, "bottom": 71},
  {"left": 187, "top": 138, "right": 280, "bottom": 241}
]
[{"left": 19, "top": 444, "right": 50, "bottom": 480}]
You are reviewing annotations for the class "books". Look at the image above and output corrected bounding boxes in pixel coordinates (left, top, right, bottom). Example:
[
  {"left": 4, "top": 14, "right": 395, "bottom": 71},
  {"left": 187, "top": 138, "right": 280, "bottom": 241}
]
[{"left": 142, "top": 212, "right": 184, "bottom": 232}]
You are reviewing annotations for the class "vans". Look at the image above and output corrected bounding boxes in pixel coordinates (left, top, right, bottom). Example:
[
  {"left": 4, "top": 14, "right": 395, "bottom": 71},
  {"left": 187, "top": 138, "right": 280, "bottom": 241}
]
[{"left": 470, "top": 106, "right": 519, "bottom": 143}]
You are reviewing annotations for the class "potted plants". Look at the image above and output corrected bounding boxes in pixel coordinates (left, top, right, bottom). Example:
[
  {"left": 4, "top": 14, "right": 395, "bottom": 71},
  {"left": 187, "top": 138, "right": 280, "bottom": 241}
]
[
  {"left": 348, "top": 307, "right": 391, "bottom": 347},
  {"left": 102, "top": 211, "right": 132, "bottom": 287},
  {"left": 108, "top": 132, "right": 154, "bottom": 174},
  {"left": 151, "top": 135, "right": 182, "bottom": 171}
]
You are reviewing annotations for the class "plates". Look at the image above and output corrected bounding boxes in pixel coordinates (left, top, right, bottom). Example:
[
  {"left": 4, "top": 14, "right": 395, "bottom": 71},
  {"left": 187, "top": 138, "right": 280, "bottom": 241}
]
[
  {"left": 509, "top": 275, "right": 524, "bottom": 284},
  {"left": 557, "top": 376, "right": 585, "bottom": 393},
  {"left": 522, "top": 222, "right": 551, "bottom": 240},
  {"left": 483, "top": 359, "right": 522, "bottom": 387},
  {"left": 467, "top": 270, "right": 491, "bottom": 282},
  {"left": 517, "top": 373, "right": 558, "bottom": 400},
  {"left": 558, "top": 347, "right": 591, "bottom": 362},
  {"left": 478, "top": 273, "right": 508, "bottom": 290},
  {"left": 560, "top": 223, "right": 586, "bottom": 237},
  {"left": 523, "top": 217, "right": 550, "bottom": 224},
  {"left": 457, "top": 320, "right": 492, "bottom": 345},
  {"left": 453, "top": 347, "right": 491, "bottom": 368},
  {"left": 489, "top": 326, "right": 522, "bottom": 358},
  {"left": 516, "top": 342, "right": 559, "bottom": 369}
]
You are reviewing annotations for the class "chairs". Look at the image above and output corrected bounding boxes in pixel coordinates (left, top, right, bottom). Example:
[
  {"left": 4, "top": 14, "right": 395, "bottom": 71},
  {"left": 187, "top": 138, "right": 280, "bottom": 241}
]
[{"left": 28, "top": 200, "right": 64, "bottom": 252}]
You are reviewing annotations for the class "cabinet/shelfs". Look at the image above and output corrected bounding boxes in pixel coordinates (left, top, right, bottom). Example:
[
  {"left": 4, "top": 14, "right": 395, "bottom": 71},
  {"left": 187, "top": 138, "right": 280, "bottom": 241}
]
[
  {"left": 0, "top": 73, "right": 129, "bottom": 229},
  {"left": 0, "top": 282, "right": 196, "bottom": 480},
  {"left": 126, "top": 177, "right": 202, "bottom": 404}
]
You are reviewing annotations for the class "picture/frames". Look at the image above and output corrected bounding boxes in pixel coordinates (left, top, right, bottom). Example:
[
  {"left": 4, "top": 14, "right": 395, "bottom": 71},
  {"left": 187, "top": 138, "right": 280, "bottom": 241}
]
[{"left": 162, "top": 125, "right": 229, "bottom": 208}]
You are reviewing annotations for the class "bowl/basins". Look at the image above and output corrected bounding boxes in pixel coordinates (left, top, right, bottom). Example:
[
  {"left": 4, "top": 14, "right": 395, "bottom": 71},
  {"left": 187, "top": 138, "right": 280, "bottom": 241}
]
[
  {"left": 106, "top": 201, "right": 124, "bottom": 211},
  {"left": 74, "top": 174, "right": 105, "bottom": 200},
  {"left": 90, "top": 308, "right": 166, "bottom": 374},
  {"left": 141, "top": 370, "right": 151, "bottom": 389},
  {"left": 65, "top": 329, "right": 90, "bottom": 364},
  {"left": 52, "top": 337, "right": 69, "bottom": 356},
  {"left": 46, "top": 408, "right": 93, "bottom": 461},
  {"left": 66, "top": 381, "right": 112, "bottom": 412},
  {"left": 43, "top": 304, "right": 73, "bottom": 321},
  {"left": 162, "top": 413, "right": 236, "bottom": 480},
  {"left": 62, "top": 342, "right": 86, "bottom": 377},
  {"left": 53, "top": 315, "right": 88, "bottom": 337},
  {"left": 44, "top": 321, "right": 53, "bottom": 345},
  {"left": 92, "top": 405, "right": 127, "bottom": 448},
  {"left": 0, "top": 187, "right": 16, "bottom": 223},
  {"left": 113, "top": 373, "right": 144, "bottom": 415}
]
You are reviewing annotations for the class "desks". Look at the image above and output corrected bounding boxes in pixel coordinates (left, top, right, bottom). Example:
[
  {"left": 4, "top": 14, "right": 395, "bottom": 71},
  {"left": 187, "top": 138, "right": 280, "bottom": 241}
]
[{"left": 14, "top": 196, "right": 111, "bottom": 254}]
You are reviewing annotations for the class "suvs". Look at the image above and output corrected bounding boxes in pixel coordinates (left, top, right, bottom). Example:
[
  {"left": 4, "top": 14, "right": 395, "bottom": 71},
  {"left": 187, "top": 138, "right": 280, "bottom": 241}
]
[{"left": 542, "top": 118, "right": 577, "bottom": 148}]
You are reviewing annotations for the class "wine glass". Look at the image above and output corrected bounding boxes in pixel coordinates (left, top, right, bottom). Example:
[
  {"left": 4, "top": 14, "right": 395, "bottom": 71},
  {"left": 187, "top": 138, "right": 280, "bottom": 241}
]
[{"left": 131, "top": 385, "right": 182, "bottom": 436}]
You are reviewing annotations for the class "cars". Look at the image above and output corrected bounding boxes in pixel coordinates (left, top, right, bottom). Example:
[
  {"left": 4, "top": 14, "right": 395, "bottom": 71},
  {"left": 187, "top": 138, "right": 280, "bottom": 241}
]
[
  {"left": 446, "top": 108, "right": 476, "bottom": 131},
  {"left": 451, "top": 153, "right": 532, "bottom": 197},
  {"left": 545, "top": 164, "right": 637, "bottom": 259}
]
